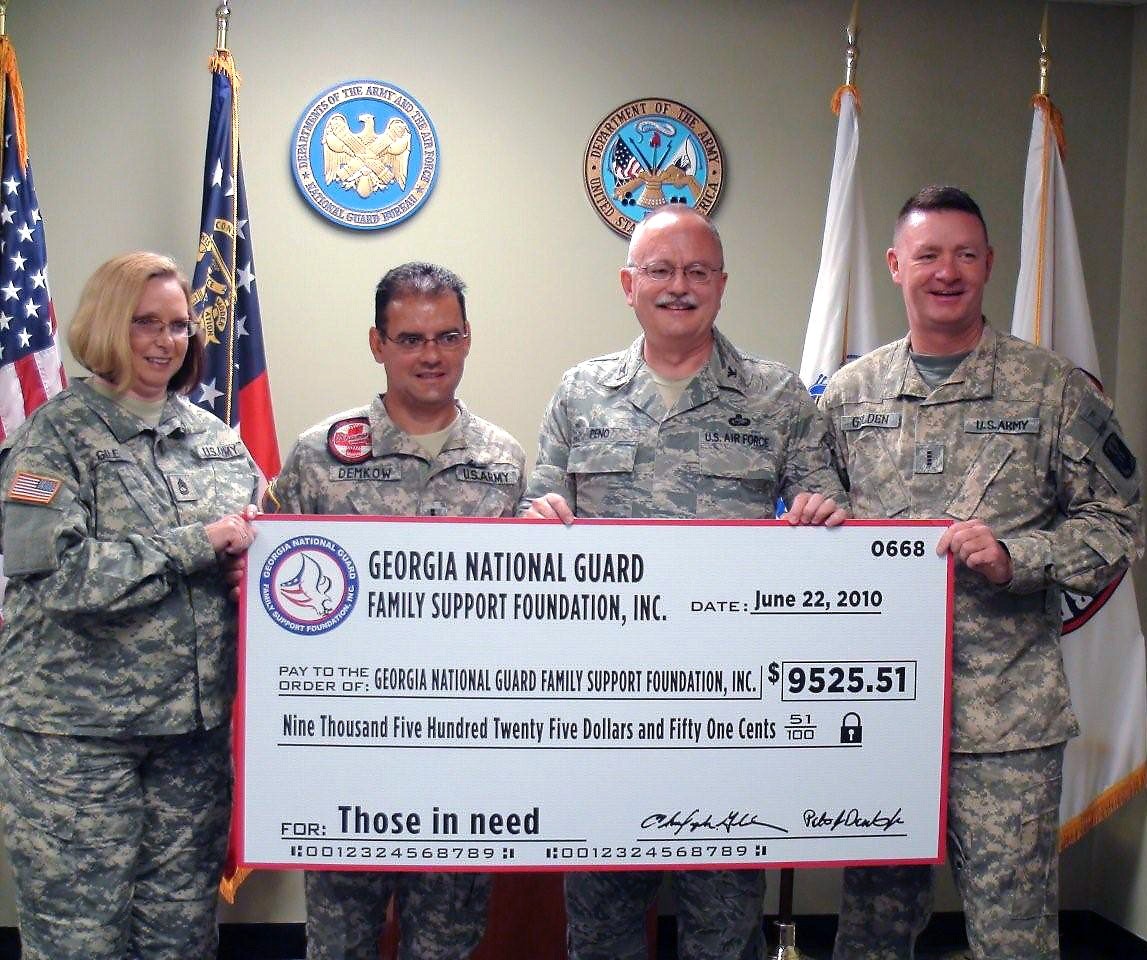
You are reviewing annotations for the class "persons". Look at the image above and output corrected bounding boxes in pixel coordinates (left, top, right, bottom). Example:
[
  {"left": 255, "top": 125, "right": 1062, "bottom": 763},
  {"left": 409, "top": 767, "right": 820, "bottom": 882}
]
[
  {"left": 816, "top": 185, "right": 1145, "bottom": 959},
  {"left": 0, "top": 252, "right": 259, "bottom": 960},
  {"left": 262, "top": 261, "right": 526, "bottom": 960},
  {"left": 514, "top": 203, "right": 854, "bottom": 959}
]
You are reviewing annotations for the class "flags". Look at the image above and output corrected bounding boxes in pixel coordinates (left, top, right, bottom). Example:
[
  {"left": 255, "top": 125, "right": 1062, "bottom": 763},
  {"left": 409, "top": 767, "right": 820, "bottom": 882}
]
[
  {"left": 0, "top": 37, "right": 68, "bottom": 446},
  {"left": 1009, "top": 94, "right": 1146, "bottom": 853},
  {"left": 188, "top": 47, "right": 282, "bottom": 907},
  {"left": 774, "top": 82, "right": 880, "bottom": 522}
]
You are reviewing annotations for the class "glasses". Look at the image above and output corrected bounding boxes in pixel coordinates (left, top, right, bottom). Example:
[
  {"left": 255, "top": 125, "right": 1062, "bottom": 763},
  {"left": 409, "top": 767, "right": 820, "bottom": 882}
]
[
  {"left": 378, "top": 323, "right": 469, "bottom": 353},
  {"left": 631, "top": 262, "right": 721, "bottom": 285},
  {"left": 129, "top": 317, "right": 199, "bottom": 340}
]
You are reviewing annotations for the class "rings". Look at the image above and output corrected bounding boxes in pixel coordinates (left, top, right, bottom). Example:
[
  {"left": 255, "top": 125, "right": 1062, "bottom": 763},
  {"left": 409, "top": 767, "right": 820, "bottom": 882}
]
[{"left": 242, "top": 534, "right": 248, "bottom": 539}]
